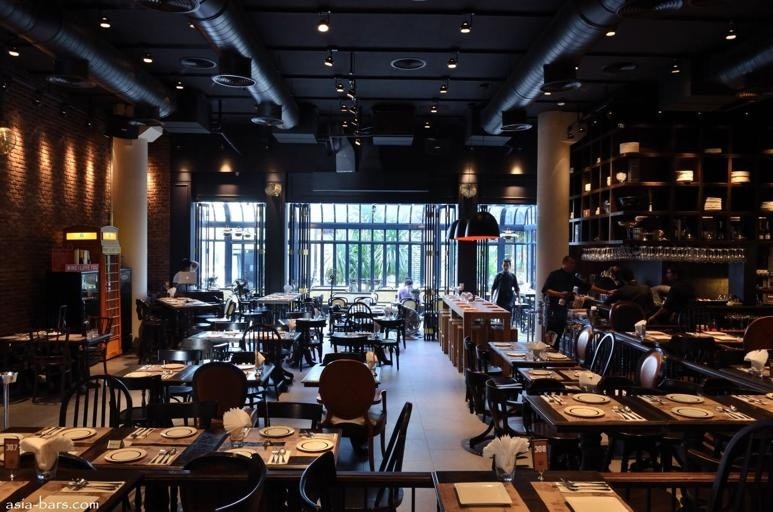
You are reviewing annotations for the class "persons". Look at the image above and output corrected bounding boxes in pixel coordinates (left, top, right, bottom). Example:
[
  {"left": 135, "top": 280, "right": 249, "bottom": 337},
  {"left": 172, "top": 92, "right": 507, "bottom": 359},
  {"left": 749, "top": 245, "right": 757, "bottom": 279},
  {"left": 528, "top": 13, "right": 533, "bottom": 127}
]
[
  {"left": 600, "top": 266, "right": 655, "bottom": 327},
  {"left": 489, "top": 259, "right": 521, "bottom": 328},
  {"left": 398, "top": 279, "right": 422, "bottom": 338},
  {"left": 645, "top": 263, "right": 695, "bottom": 330},
  {"left": 539, "top": 256, "right": 614, "bottom": 352}
]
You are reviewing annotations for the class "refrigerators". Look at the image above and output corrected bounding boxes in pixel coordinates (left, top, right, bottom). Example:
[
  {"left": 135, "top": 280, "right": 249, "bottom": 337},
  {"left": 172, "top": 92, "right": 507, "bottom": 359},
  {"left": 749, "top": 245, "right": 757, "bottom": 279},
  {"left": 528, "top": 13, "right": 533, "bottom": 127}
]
[{"left": 44, "top": 270, "right": 101, "bottom": 366}]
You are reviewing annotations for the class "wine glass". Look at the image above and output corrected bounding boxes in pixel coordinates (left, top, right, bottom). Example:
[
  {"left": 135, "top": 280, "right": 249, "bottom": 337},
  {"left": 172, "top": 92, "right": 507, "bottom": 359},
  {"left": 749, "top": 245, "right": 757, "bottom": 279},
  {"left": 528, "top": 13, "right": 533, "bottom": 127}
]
[{"left": 580, "top": 245, "right": 746, "bottom": 263}]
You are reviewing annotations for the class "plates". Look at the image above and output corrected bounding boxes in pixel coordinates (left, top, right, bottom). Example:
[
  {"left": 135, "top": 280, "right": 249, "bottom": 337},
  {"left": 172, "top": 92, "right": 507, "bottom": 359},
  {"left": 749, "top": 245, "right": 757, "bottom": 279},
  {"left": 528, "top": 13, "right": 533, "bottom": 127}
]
[
  {"left": 671, "top": 407, "right": 714, "bottom": 418},
  {"left": 59, "top": 427, "right": 96, "bottom": 439},
  {"left": 124, "top": 363, "right": 184, "bottom": 378},
  {"left": 454, "top": 292, "right": 506, "bottom": 314},
  {"left": 104, "top": 447, "right": 147, "bottom": 462},
  {"left": 259, "top": 425, "right": 295, "bottom": 437},
  {"left": 223, "top": 448, "right": 257, "bottom": 460},
  {"left": 26, "top": 494, "right": 99, "bottom": 511},
  {"left": 159, "top": 426, "right": 197, "bottom": 439},
  {"left": 453, "top": 482, "right": 513, "bottom": 507},
  {"left": 562, "top": 406, "right": 607, "bottom": 419},
  {"left": 0, "top": 432, "right": 24, "bottom": 463},
  {"left": 295, "top": 438, "right": 335, "bottom": 453},
  {"left": 565, "top": 496, "right": 630, "bottom": 512},
  {"left": 665, "top": 392, "right": 705, "bottom": 404},
  {"left": 573, "top": 393, "right": 610, "bottom": 406},
  {"left": 493, "top": 339, "right": 595, "bottom": 382}
]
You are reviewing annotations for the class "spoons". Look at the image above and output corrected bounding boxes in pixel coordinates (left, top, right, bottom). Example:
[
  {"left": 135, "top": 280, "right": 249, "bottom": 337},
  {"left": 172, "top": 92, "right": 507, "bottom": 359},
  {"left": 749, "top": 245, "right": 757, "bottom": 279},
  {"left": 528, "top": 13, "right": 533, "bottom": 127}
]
[
  {"left": 62, "top": 472, "right": 122, "bottom": 492},
  {"left": 148, "top": 446, "right": 177, "bottom": 466},
  {"left": 557, "top": 473, "right": 612, "bottom": 493},
  {"left": 267, "top": 447, "right": 287, "bottom": 464},
  {"left": 612, "top": 404, "right": 644, "bottom": 421},
  {"left": 544, "top": 392, "right": 567, "bottom": 406},
  {"left": 715, "top": 404, "right": 751, "bottom": 419}
]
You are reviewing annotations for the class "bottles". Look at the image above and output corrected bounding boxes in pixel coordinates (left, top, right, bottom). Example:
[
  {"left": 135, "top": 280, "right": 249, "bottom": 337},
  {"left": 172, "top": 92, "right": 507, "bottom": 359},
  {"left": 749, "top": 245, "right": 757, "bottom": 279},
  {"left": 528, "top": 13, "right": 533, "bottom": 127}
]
[
  {"left": 694, "top": 294, "right": 751, "bottom": 338},
  {"left": 756, "top": 220, "right": 772, "bottom": 242}
]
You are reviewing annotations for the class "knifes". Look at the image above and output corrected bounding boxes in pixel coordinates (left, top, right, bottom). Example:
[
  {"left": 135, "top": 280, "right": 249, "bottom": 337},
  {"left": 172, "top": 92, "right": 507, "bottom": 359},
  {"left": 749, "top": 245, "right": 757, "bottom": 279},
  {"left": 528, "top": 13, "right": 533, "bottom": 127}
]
[{"left": 34, "top": 424, "right": 65, "bottom": 439}]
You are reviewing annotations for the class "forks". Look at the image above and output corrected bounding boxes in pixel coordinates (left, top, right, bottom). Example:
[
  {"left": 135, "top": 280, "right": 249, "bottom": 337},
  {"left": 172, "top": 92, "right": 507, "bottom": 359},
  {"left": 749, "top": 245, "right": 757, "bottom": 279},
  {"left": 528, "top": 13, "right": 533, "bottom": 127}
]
[{"left": 130, "top": 426, "right": 153, "bottom": 439}]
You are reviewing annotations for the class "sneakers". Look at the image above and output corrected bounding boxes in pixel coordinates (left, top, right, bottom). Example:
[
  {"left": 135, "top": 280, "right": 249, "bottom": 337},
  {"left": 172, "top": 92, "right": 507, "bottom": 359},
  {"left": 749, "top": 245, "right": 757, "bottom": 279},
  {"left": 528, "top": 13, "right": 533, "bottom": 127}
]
[{"left": 415, "top": 331, "right": 421, "bottom": 336}]
